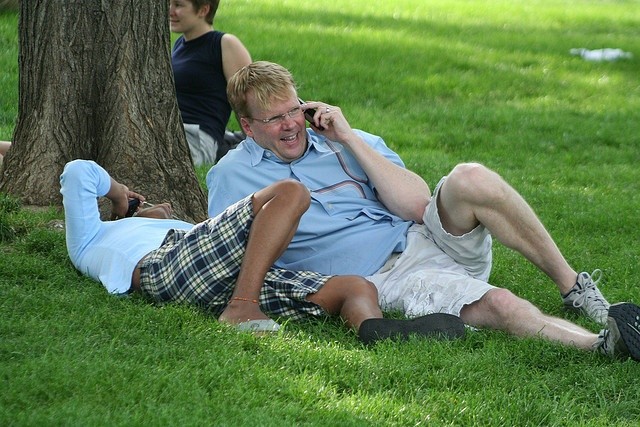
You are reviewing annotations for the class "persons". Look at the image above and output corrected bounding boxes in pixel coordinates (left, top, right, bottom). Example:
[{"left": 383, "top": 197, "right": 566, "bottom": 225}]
[
  {"left": 59, "top": 159, "right": 465, "bottom": 348},
  {"left": 206, "top": 61, "right": 640, "bottom": 361},
  {"left": 170, "top": 0, "right": 252, "bottom": 168}
]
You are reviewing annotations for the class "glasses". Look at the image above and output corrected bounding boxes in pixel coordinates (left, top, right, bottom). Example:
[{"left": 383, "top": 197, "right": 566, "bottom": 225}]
[{"left": 245, "top": 105, "right": 305, "bottom": 124}]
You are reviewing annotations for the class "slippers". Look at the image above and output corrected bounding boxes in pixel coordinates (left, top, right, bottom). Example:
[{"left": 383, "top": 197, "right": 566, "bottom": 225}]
[
  {"left": 238, "top": 318, "right": 282, "bottom": 335},
  {"left": 359, "top": 314, "right": 464, "bottom": 343}
]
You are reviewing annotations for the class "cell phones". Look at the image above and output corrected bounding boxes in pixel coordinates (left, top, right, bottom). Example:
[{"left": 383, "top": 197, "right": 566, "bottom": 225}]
[
  {"left": 298, "top": 97, "right": 332, "bottom": 131},
  {"left": 112, "top": 197, "right": 140, "bottom": 219}
]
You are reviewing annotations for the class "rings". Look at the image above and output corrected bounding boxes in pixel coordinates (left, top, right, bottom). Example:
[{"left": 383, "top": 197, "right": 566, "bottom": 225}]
[{"left": 326, "top": 108, "right": 330, "bottom": 113}]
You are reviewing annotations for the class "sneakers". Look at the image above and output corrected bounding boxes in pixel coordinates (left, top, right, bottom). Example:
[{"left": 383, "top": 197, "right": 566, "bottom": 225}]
[
  {"left": 561, "top": 269, "right": 612, "bottom": 328},
  {"left": 592, "top": 301, "right": 640, "bottom": 361}
]
